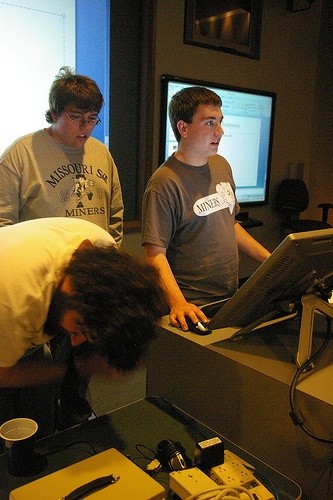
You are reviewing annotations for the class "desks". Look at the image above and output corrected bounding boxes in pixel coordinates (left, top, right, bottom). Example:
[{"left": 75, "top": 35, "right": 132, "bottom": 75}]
[
  {"left": 0, "top": 394, "right": 303, "bottom": 500},
  {"left": 237, "top": 219, "right": 333, "bottom": 280}
]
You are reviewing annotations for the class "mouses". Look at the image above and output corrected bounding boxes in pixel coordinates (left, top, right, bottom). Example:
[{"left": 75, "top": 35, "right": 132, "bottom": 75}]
[
  {"left": 181, "top": 315, "right": 212, "bottom": 336},
  {"left": 158, "top": 440, "right": 188, "bottom": 472}
]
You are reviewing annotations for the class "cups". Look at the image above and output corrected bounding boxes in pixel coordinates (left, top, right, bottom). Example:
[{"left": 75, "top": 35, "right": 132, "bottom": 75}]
[{"left": 0, "top": 418, "right": 38, "bottom": 477}]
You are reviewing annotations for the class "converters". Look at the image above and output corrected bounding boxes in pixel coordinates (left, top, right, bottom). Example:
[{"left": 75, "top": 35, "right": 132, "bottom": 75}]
[{"left": 168, "top": 467, "right": 223, "bottom": 500}]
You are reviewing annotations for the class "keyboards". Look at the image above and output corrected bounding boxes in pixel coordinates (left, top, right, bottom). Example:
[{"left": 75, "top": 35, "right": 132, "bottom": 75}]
[{"left": 200, "top": 297, "right": 231, "bottom": 318}]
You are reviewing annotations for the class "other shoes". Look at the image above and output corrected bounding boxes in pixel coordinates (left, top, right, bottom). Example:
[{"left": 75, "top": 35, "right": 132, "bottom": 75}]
[{"left": 61, "top": 396, "right": 92, "bottom": 421}]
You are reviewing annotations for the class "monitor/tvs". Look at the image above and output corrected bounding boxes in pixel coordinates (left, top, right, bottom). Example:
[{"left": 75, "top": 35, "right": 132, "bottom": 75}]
[
  {"left": 158, "top": 73, "right": 277, "bottom": 229},
  {"left": 207, "top": 229, "right": 333, "bottom": 331}
]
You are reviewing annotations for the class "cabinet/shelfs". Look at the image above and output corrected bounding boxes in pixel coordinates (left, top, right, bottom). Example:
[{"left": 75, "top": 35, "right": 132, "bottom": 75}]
[{"left": 146, "top": 298, "right": 333, "bottom": 500}]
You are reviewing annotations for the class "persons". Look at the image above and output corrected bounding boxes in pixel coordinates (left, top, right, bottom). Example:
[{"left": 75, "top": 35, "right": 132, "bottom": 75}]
[
  {"left": 0, "top": 218, "right": 164, "bottom": 436},
  {"left": 0, "top": 66, "right": 123, "bottom": 251},
  {"left": 141, "top": 87, "right": 272, "bottom": 330}
]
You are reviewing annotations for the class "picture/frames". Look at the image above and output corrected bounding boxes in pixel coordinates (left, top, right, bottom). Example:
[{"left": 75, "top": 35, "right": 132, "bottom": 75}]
[{"left": 183, "top": 0, "right": 264, "bottom": 60}]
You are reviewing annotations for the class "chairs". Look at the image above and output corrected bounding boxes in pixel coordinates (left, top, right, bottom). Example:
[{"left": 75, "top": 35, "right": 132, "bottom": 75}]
[{"left": 273, "top": 179, "right": 333, "bottom": 231}]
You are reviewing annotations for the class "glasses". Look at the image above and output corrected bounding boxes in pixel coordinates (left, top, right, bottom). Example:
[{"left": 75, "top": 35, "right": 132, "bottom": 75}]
[{"left": 62, "top": 108, "right": 101, "bottom": 127}]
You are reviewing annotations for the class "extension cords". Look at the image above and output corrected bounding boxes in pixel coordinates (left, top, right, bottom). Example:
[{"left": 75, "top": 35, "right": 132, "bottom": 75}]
[{"left": 211, "top": 460, "right": 275, "bottom": 500}]
